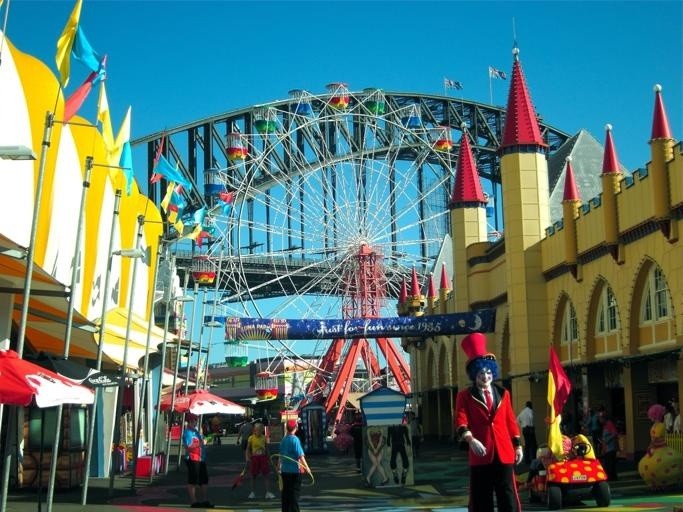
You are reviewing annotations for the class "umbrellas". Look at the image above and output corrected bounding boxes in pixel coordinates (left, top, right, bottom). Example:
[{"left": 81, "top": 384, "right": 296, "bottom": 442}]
[
  {"left": 1, "top": 350, "right": 95, "bottom": 409},
  {"left": 159, "top": 387, "right": 247, "bottom": 433}
]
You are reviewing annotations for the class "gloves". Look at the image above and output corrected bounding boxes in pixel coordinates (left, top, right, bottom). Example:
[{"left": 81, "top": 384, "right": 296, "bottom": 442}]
[
  {"left": 469, "top": 438, "right": 487, "bottom": 457},
  {"left": 515, "top": 447, "right": 523, "bottom": 465}
]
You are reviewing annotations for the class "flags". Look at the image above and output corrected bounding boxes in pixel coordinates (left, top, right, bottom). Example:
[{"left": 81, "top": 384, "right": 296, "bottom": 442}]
[
  {"left": 490, "top": 66, "right": 506, "bottom": 79},
  {"left": 546, "top": 347, "right": 572, "bottom": 414},
  {"left": 55, "top": 0, "right": 210, "bottom": 250},
  {"left": 445, "top": 79, "right": 462, "bottom": 88}
]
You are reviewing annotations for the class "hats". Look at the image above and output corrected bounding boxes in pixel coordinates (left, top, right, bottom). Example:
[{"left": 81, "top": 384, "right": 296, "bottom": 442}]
[
  {"left": 287, "top": 418, "right": 298, "bottom": 428},
  {"left": 186, "top": 413, "right": 199, "bottom": 421},
  {"left": 460, "top": 332, "right": 497, "bottom": 367}
]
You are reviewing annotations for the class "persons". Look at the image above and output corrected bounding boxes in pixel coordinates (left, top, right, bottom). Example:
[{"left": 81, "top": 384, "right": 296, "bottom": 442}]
[
  {"left": 544, "top": 402, "right": 618, "bottom": 481},
  {"left": 327, "top": 410, "right": 363, "bottom": 472},
  {"left": 454, "top": 357, "right": 525, "bottom": 511},
  {"left": 646, "top": 398, "right": 681, "bottom": 443},
  {"left": 386, "top": 422, "right": 411, "bottom": 484},
  {"left": 403, "top": 410, "right": 425, "bottom": 446},
  {"left": 366, "top": 425, "right": 390, "bottom": 487},
  {"left": 516, "top": 400, "right": 538, "bottom": 464},
  {"left": 183, "top": 413, "right": 310, "bottom": 511}
]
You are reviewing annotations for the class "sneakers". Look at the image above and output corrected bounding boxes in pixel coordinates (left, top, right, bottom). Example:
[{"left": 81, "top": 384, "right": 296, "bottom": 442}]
[
  {"left": 191, "top": 502, "right": 215, "bottom": 508},
  {"left": 248, "top": 492, "right": 256, "bottom": 498},
  {"left": 265, "top": 492, "right": 276, "bottom": 498}
]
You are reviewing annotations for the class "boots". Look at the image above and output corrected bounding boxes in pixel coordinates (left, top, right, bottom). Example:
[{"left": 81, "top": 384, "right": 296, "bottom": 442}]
[
  {"left": 393, "top": 471, "right": 400, "bottom": 484},
  {"left": 401, "top": 469, "right": 408, "bottom": 484}
]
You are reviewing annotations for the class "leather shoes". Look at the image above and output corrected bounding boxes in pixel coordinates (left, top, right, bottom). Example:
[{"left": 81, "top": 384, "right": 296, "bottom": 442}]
[
  {"left": 364, "top": 477, "right": 372, "bottom": 487},
  {"left": 380, "top": 477, "right": 389, "bottom": 486}
]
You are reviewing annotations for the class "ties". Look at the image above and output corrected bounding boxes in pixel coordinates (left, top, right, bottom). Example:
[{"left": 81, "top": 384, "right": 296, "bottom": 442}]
[{"left": 484, "top": 388, "right": 495, "bottom": 416}]
